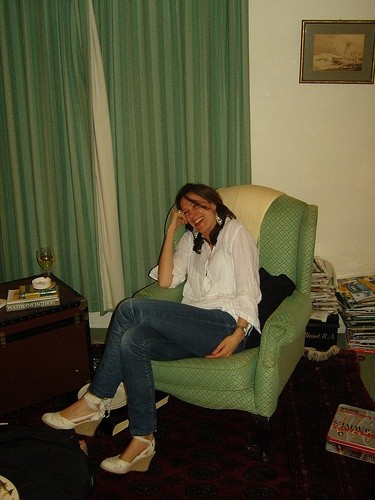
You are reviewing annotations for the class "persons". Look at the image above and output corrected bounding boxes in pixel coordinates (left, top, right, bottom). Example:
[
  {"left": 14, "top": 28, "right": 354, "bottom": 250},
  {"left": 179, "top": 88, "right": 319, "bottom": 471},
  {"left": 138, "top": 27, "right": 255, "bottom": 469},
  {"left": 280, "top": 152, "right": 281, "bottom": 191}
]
[{"left": 42, "top": 182, "right": 262, "bottom": 474}]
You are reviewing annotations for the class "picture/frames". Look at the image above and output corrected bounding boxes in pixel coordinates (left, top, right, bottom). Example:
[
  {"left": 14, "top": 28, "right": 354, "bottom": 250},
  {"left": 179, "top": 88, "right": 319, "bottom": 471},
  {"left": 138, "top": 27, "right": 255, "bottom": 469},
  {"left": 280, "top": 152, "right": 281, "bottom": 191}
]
[{"left": 298, "top": 20, "right": 375, "bottom": 84}]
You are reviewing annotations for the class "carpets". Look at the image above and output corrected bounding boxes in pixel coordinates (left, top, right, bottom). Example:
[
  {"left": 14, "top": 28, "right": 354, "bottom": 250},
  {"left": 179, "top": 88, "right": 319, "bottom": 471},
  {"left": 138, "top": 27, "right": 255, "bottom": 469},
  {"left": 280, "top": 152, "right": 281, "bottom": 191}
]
[{"left": 0, "top": 343, "right": 375, "bottom": 500}]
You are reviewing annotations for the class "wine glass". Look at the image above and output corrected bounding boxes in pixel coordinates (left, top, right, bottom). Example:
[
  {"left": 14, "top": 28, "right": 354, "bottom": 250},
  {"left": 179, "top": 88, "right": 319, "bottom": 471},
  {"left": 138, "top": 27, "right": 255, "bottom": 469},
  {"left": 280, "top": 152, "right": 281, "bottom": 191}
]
[{"left": 36, "top": 247, "right": 56, "bottom": 277}]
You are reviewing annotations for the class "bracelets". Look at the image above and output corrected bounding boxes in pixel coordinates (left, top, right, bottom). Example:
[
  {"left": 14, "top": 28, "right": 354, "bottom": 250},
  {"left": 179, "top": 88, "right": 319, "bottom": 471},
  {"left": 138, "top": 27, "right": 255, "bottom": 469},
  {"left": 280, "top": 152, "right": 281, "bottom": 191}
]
[{"left": 236, "top": 324, "right": 248, "bottom": 336}]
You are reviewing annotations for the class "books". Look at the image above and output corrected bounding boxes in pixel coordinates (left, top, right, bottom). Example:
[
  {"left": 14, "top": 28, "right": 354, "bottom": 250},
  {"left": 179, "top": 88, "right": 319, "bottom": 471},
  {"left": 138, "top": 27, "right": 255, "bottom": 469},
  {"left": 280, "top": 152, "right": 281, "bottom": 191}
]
[
  {"left": 310, "top": 255, "right": 375, "bottom": 354},
  {"left": 6, "top": 282, "right": 60, "bottom": 312}
]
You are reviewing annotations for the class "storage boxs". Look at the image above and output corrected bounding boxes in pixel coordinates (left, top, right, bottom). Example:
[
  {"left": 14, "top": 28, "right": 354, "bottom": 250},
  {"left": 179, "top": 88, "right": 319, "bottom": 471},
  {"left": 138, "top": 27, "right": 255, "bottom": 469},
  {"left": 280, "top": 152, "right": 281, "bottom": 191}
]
[
  {"left": 304, "top": 314, "right": 340, "bottom": 352},
  {"left": 325, "top": 405, "right": 375, "bottom": 465}
]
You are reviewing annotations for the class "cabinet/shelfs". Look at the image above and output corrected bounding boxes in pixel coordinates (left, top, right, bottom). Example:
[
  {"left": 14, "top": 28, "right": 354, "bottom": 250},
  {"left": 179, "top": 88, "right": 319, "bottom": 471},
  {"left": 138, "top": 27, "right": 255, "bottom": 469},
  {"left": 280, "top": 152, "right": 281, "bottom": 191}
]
[{"left": 0, "top": 272, "right": 94, "bottom": 418}]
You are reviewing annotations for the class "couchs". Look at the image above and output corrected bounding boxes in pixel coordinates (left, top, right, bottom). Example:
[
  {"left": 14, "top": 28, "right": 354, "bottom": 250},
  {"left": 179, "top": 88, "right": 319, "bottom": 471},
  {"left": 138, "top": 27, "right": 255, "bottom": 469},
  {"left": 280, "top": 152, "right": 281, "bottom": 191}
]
[{"left": 134, "top": 185, "right": 318, "bottom": 430}]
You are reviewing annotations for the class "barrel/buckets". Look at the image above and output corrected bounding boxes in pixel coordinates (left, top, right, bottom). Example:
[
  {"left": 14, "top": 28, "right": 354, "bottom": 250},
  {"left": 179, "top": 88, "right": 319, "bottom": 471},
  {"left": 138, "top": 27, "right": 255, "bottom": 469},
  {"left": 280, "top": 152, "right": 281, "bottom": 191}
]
[{"left": 77, "top": 382, "right": 129, "bottom": 439}]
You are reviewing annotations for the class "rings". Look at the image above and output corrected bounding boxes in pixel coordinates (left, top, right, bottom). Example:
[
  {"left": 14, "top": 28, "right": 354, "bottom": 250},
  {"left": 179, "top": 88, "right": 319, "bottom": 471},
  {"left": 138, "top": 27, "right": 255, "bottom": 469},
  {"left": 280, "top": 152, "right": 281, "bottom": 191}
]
[{"left": 178, "top": 210, "right": 181, "bottom": 213}]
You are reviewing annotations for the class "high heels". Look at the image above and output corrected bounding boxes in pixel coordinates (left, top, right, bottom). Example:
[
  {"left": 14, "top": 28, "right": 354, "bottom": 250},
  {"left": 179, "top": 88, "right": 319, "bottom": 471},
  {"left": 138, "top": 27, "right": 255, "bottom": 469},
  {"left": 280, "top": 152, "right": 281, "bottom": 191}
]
[
  {"left": 101, "top": 434, "right": 156, "bottom": 474},
  {"left": 42, "top": 393, "right": 111, "bottom": 438}
]
